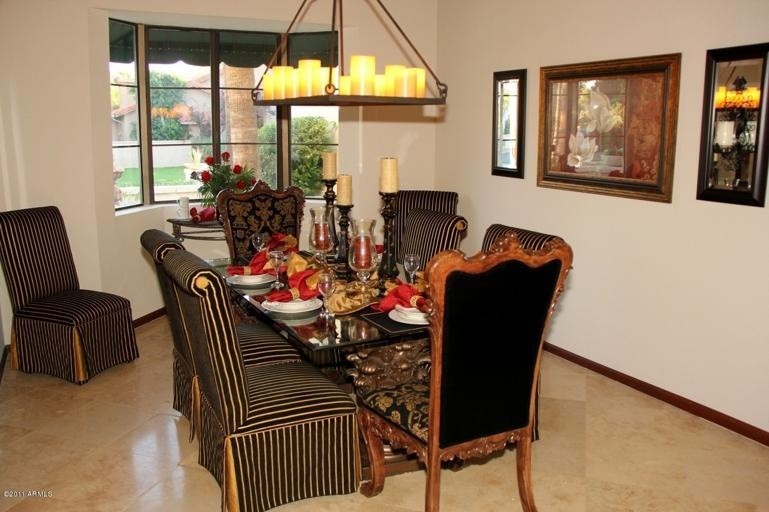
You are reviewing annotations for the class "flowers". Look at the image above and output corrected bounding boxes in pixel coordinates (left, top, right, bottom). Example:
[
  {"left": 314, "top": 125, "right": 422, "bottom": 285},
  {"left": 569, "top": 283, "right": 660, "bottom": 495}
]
[{"left": 191, "top": 153, "right": 256, "bottom": 207}]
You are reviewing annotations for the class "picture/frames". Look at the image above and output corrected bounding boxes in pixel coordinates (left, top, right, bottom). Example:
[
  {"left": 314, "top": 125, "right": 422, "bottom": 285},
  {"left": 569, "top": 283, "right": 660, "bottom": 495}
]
[
  {"left": 696, "top": 42, "right": 769, "bottom": 208},
  {"left": 537, "top": 53, "right": 682, "bottom": 202},
  {"left": 491, "top": 69, "right": 527, "bottom": 179}
]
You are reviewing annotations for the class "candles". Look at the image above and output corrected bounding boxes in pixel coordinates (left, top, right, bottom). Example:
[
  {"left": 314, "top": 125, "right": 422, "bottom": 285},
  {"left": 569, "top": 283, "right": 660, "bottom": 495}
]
[
  {"left": 336, "top": 174, "right": 353, "bottom": 205},
  {"left": 715, "top": 120, "right": 735, "bottom": 147},
  {"left": 323, "top": 152, "right": 336, "bottom": 180},
  {"left": 379, "top": 157, "right": 398, "bottom": 192}
]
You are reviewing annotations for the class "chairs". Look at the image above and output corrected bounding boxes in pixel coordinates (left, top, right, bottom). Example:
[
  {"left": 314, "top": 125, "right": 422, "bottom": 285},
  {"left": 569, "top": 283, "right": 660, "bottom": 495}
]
[
  {"left": 140, "top": 179, "right": 572, "bottom": 512},
  {"left": 0, "top": 205, "right": 140, "bottom": 385}
]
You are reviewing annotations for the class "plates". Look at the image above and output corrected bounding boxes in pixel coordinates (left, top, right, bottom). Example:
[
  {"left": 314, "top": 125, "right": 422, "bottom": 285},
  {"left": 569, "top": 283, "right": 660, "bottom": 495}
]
[
  {"left": 225, "top": 273, "right": 276, "bottom": 289},
  {"left": 387, "top": 304, "right": 431, "bottom": 325},
  {"left": 261, "top": 298, "right": 323, "bottom": 314}
]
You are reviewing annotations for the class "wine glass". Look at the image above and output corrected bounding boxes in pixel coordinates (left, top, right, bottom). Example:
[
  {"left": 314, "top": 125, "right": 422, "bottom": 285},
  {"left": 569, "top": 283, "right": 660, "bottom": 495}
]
[
  {"left": 404, "top": 253, "right": 421, "bottom": 284},
  {"left": 309, "top": 207, "right": 335, "bottom": 268},
  {"left": 269, "top": 251, "right": 284, "bottom": 287},
  {"left": 348, "top": 217, "right": 379, "bottom": 282},
  {"left": 318, "top": 274, "right": 335, "bottom": 318}
]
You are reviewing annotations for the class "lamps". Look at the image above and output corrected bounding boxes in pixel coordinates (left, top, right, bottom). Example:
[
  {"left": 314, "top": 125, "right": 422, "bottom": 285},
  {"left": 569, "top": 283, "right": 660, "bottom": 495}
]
[
  {"left": 251, "top": 0, "right": 447, "bottom": 106},
  {"left": 715, "top": 77, "right": 761, "bottom": 109}
]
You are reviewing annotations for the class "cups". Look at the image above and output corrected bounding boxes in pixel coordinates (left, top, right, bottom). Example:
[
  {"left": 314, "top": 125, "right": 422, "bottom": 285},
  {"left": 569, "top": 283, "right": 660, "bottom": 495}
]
[
  {"left": 176, "top": 196, "right": 190, "bottom": 219},
  {"left": 251, "top": 233, "right": 269, "bottom": 252}
]
